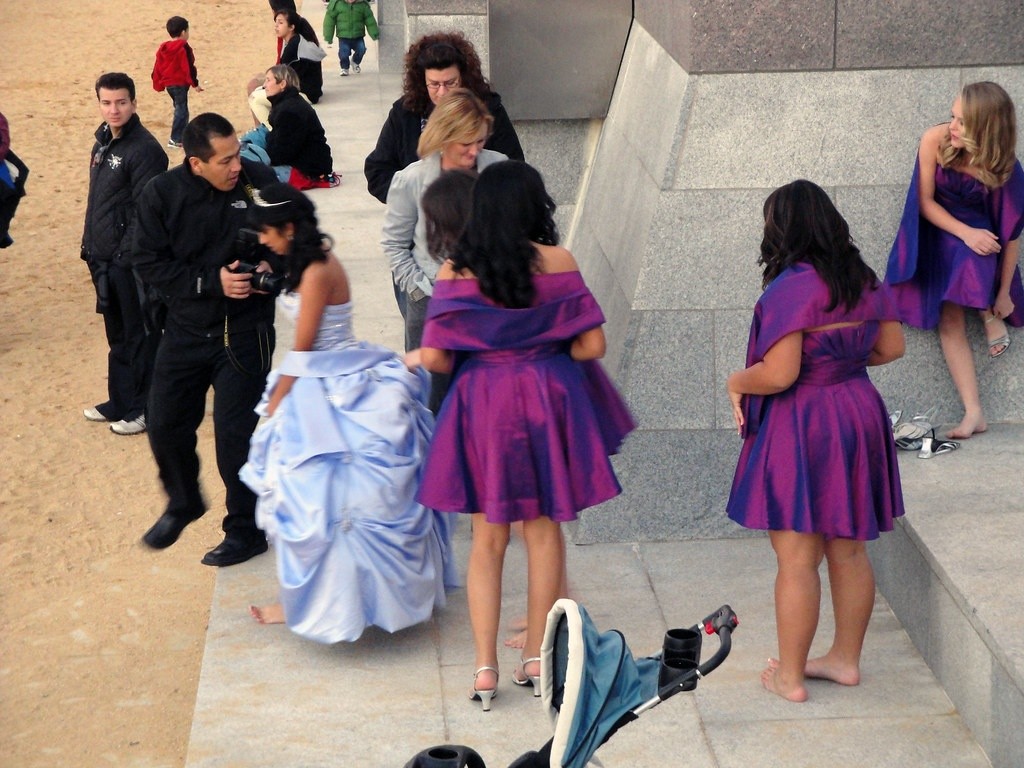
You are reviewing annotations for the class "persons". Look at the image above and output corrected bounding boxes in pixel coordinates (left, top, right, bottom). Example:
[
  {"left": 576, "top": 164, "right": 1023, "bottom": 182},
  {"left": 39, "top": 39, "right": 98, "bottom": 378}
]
[
  {"left": 151, "top": 16, "right": 204, "bottom": 148},
  {"left": 729, "top": 178, "right": 907, "bottom": 704},
  {"left": 141, "top": 113, "right": 280, "bottom": 565},
  {"left": 382, "top": 86, "right": 515, "bottom": 418},
  {"left": 81, "top": 73, "right": 168, "bottom": 434},
  {"left": 323, "top": 0, "right": 380, "bottom": 76},
  {"left": 240, "top": 0, "right": 333, "bottom": 177},
  {"left": 405, "top": 161, "right": 624, "bottom": 710},
  {"left": 883, "top": 80, "right": 1024, "bottom": 437},
  {"left": 246, "top": 183, "right": 464, "bottom": 645},
  {"left": 365, "top": 31, "right": 525, "bottom": 320}
]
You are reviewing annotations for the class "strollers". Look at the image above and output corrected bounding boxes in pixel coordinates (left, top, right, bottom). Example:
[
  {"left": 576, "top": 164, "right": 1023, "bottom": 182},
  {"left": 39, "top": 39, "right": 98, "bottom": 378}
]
[{"left": 401, "top": 598, "right": 739, "bottom": 768}]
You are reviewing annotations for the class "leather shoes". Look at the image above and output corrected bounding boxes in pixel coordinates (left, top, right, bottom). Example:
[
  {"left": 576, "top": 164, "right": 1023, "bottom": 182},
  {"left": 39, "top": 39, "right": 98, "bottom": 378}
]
[
  {"left": 144, "top": 503, "right": 206, "bottom": 549},
  {"left": 201, "top": 532, "right": 268, "bottom": 567}
]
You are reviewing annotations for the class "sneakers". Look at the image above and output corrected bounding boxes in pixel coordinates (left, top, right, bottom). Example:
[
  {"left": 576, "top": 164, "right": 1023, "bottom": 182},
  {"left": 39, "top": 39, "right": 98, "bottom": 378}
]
[
  {"left": 84, "top": 408, "right": 106, "bottom": 422},
  {"left": 109, "top": 409, "right": 148, "bottom": 435}
]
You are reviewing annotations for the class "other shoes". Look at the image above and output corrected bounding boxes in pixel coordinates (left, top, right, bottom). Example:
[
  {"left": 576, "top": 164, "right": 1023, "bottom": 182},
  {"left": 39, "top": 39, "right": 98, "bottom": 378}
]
[
  {"left": 340, "top": 68, "right": 350, "bottom": 76},
  {"left": 167, "top": 139, "right": 184, "bottom": 150},
  {"left": 351, "top": 56, "right": 361, "bottom": 73}
]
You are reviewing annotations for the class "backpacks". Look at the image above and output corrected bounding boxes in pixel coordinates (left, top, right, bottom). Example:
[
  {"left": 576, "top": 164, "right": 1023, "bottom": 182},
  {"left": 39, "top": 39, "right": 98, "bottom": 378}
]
[{"left": 240, "top": 141, "right": 271, "bottom": 167}]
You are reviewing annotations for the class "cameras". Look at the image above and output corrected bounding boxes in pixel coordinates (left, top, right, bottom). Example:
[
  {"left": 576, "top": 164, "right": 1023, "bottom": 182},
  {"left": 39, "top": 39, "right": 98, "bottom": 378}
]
[{"left": 230, "top": 228, "right": 284, "bottom": 296}]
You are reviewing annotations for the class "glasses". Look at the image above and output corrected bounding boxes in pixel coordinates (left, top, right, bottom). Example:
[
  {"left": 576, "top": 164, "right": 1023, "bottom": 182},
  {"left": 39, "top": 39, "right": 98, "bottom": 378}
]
[{"left": 420, "top": 76, "right": 460, "bottom": 90}]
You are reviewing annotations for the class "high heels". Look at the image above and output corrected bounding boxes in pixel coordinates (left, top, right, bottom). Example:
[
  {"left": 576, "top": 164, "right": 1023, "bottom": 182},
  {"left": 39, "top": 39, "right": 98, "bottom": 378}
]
[
  {"left": 981, "top": 312, "right": 1011, "bottom": 357},
  {"left": 511, "top": 657, "right": 543, "bottom": 699},
  {"left": 889, "top": 405, "right": 957, "bottom": 458},
  {"left": 467, "top": 666, "right": 500, "bottom": 712}
]
